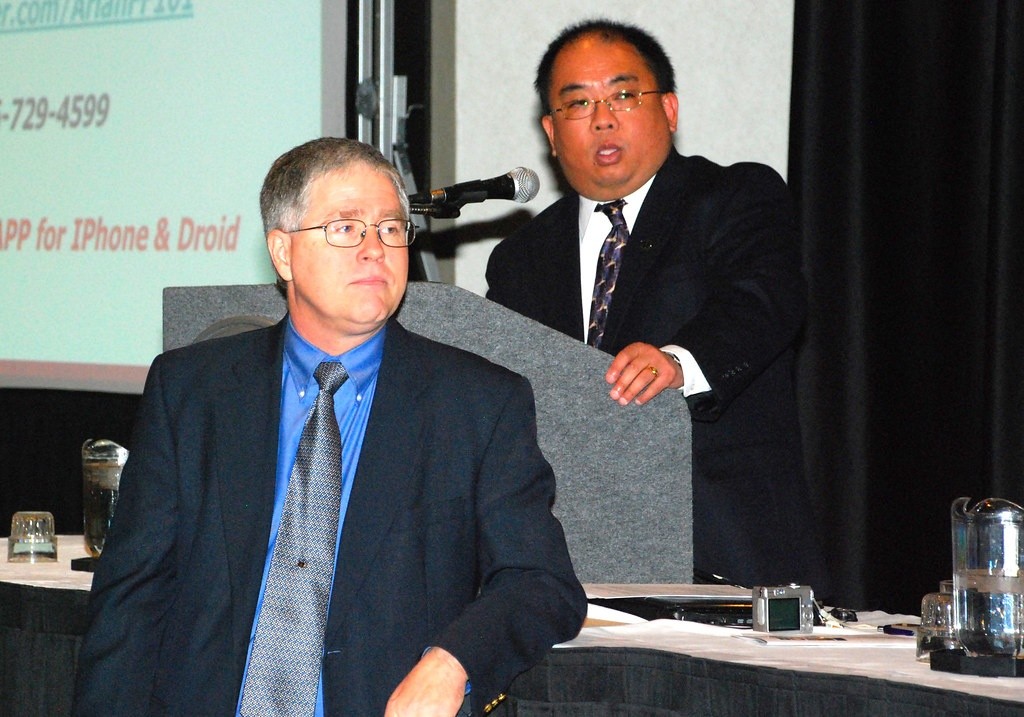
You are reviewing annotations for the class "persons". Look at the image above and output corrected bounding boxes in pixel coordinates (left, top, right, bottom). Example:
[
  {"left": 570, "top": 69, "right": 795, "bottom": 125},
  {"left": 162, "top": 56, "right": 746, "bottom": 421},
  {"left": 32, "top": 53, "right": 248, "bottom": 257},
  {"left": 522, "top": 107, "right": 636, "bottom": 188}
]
[
  {"left": 73, "top": 138, "right": 589, "bottom": 717},
  {"left": 484, "top": 21, "right": 822, "bottom": 589}
]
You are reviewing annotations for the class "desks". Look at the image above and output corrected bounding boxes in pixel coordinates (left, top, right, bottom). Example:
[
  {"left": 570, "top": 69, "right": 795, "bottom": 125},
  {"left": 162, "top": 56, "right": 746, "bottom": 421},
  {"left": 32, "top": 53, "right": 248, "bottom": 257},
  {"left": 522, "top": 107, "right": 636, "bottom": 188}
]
[{"left": 0, "top": 533, "right": 1024, "bottom": 717}]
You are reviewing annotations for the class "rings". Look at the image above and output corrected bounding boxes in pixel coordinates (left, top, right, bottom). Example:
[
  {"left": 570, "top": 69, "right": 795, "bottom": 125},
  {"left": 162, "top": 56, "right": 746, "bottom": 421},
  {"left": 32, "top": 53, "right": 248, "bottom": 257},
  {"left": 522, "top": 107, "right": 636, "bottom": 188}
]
[{"left": 647, "top": 366, "right": 658, "bottom": 376}]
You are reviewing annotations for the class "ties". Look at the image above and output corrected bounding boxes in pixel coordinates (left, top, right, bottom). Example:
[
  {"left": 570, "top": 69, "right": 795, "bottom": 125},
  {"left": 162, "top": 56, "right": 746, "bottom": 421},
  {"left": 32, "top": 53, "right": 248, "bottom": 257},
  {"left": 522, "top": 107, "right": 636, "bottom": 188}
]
[
  {"left": 238, "top": 359, "right": 349, "bottom": 717},
  {"left": 587, "top": 197, "right": 631, "bottom": 348}
]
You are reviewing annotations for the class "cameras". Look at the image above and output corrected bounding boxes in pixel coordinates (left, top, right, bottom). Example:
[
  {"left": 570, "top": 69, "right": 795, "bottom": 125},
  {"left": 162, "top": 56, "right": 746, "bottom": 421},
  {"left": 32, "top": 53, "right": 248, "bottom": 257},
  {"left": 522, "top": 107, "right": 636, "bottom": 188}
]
[{"left": 751, "top": 582, "right": 814, "bottom": 634}]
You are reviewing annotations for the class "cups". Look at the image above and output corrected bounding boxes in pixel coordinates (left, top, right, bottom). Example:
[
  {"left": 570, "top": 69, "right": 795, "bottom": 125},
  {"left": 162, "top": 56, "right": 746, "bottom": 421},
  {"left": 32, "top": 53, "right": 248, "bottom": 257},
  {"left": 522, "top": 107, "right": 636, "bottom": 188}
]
[
  {"left": 916, "top": 580, "right": 959, "bottom": 664},
  {"left": 8, "top": 511, "right": 57, "bottom": 564}
]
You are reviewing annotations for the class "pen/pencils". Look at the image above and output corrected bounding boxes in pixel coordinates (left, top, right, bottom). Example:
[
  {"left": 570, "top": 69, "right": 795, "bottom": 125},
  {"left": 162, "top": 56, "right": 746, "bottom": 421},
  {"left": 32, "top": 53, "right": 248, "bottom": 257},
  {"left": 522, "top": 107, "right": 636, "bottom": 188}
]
[{"left": 877, "top": 625, "right": 915, "bottom": 636}]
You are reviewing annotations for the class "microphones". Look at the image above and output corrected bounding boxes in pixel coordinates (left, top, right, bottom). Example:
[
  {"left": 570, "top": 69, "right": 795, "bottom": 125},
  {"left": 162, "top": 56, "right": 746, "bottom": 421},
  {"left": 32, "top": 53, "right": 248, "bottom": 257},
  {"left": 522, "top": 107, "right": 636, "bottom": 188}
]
[{"left": 411, "top": 166, "right": 541, "bottom": 203}]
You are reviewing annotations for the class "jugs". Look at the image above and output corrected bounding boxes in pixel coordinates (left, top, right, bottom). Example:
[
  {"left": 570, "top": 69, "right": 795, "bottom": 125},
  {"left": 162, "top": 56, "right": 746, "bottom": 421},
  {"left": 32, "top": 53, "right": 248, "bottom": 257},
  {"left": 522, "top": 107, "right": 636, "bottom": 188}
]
[
  {"left": 83, "top": 437, "right": 128, "bottom": 559},
  {"left": 951, "top": 495, "right": 1024, "bottom": 662}
]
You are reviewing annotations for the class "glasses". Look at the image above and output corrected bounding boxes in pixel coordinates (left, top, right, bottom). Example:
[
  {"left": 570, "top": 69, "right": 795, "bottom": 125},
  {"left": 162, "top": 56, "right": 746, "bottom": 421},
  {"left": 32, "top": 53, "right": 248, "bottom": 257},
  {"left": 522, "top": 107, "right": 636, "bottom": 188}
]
[
  {"left": 283, "top": 219, "right": 421, "bottom": 248},
  {"left": 549, "top": 88, "right": 670, "bottom": 120}
]
